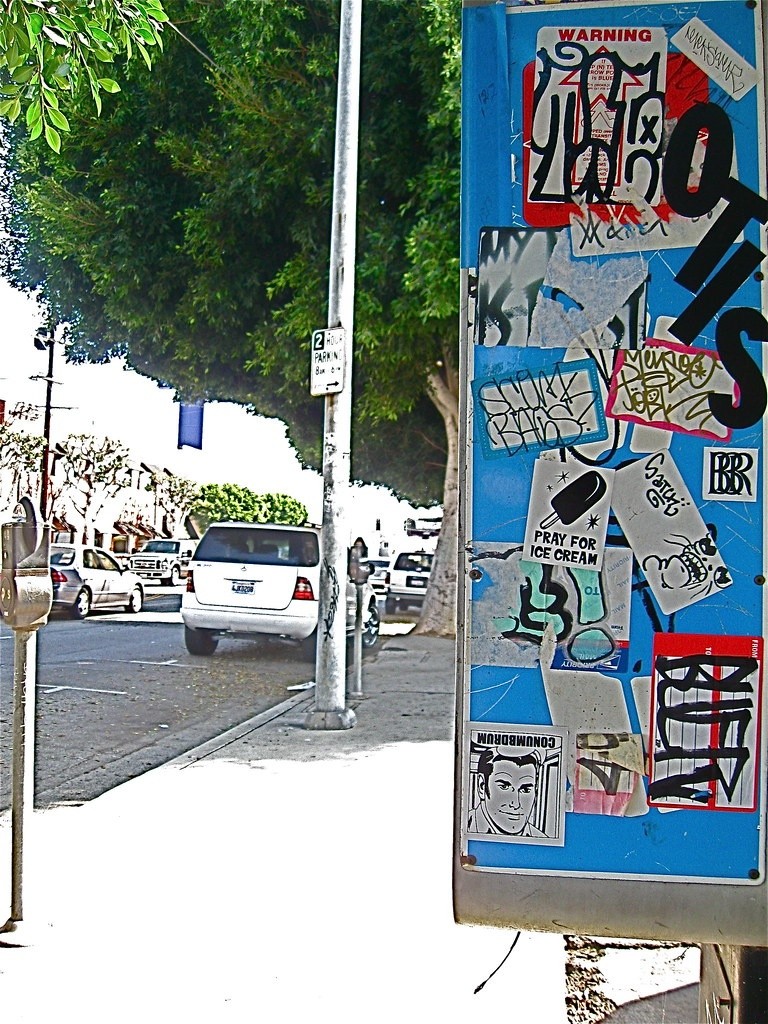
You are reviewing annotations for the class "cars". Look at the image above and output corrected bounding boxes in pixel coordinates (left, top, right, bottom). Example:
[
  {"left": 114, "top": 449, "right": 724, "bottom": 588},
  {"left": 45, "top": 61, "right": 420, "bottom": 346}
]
[
  {"left": 384, "top": 550, "right": 436, "bottom": 614},
  {"left": 48, "top": 541, "right": 147, "bottom": 617},
  {"left": 179, "top": 522, "right": 380, "bottom": 656}
]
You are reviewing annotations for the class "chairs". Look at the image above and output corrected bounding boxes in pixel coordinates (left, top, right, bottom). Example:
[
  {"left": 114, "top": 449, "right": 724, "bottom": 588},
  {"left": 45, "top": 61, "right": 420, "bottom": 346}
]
[{"left": 257, "top": 544, "right": 280, "bottom": 560}]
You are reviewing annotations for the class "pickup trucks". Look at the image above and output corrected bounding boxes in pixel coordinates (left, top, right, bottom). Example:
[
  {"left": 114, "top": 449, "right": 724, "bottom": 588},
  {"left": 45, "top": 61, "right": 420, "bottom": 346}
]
[{"left": 125, "top": 538, "right": 197, "bottom": 586}]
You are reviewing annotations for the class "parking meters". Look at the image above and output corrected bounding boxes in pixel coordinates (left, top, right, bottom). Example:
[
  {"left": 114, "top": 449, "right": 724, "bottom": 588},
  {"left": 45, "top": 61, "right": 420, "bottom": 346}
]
[
  {"left": 1, "top": 496, "right": 54, "bottom": 949},
  {"left": 348, "top": 532, "right": 376, "bottom": 699}
]
